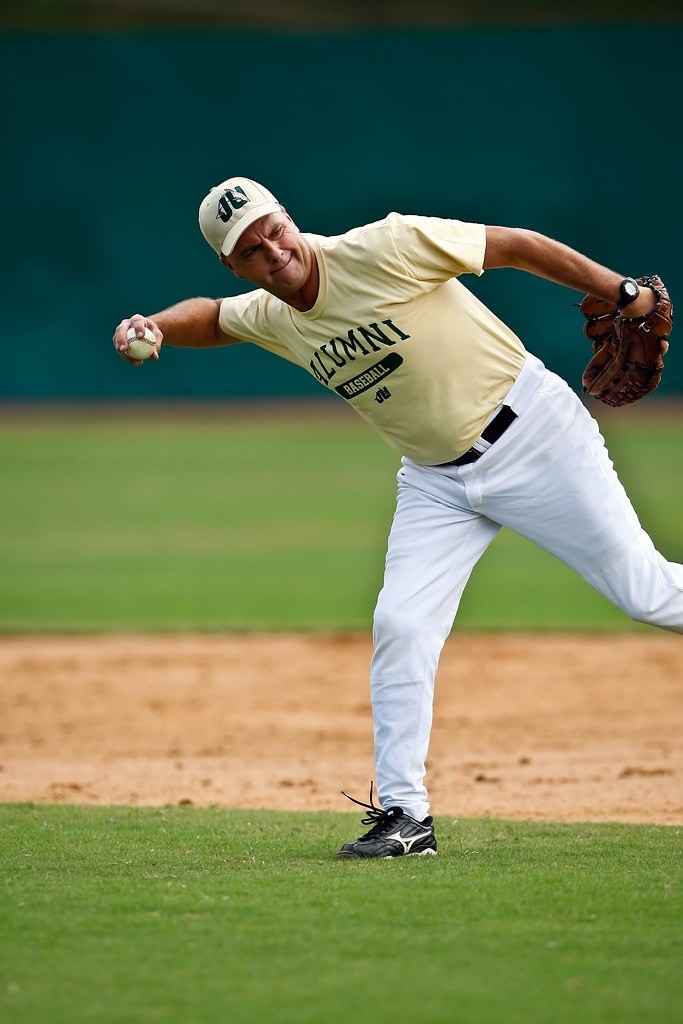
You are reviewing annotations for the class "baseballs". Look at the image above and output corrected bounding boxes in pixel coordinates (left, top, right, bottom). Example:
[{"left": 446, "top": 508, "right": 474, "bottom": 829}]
[{"left": 124, "top": 327, "right": 157, "bottom": 360}]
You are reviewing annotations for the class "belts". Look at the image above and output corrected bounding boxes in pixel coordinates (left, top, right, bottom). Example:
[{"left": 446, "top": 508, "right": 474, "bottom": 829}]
[{"left": 434, "top": 405, "right": 519, "bottom": 465}]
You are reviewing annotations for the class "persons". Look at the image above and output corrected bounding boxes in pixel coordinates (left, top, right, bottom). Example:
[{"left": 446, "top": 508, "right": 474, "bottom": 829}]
[{"left": 112, "top": 174, "right": 683, "bottom": 860}]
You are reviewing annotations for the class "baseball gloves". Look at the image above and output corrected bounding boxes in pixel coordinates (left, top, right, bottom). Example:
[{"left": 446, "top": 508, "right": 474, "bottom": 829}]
[{"left": 570, "top": 274, "right": 675, "bottom": 410}]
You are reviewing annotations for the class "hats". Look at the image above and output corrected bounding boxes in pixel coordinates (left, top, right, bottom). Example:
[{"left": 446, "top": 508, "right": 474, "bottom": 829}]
[{"left": 198, "top": 176, "right": 282, "bottom": 256}]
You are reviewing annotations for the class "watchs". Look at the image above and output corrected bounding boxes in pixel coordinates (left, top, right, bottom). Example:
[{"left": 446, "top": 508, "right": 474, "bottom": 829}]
[{"left": 614, "top": 277, "right": 639, "bottom": 310}]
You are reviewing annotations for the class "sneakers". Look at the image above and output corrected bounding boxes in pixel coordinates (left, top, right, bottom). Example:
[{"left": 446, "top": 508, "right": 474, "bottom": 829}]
[{"left": 338, "top": 780, "right": 439, "bottom": 862}]
